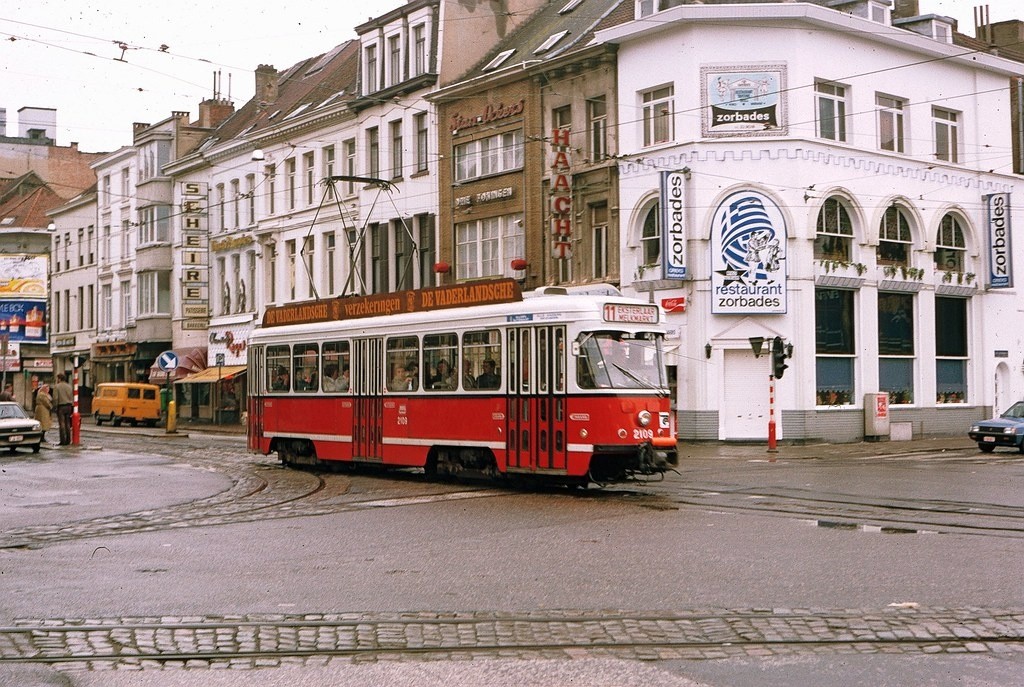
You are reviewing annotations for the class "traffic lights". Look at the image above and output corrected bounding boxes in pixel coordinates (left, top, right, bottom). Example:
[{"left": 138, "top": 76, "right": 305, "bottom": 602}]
[{"left": 772, "top": 336, "right": 788, "bottom": 379}]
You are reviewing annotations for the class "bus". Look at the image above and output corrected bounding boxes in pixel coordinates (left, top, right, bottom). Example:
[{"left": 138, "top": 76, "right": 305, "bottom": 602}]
[{"left": 245, "top": 276, "right": 681, "bottom": 494}]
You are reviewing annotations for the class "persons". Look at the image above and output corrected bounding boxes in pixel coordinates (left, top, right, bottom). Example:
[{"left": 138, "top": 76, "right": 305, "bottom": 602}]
[
  {"left": 0, "top": 384, "right": 16, "bottom": 402},
  {"left": 52, "top": 372, "right": 73, "bottom": 446},
  {"left": 32, "top": 384, "right": 53, "bottom": 442},
  {"left": 273, "top": 356, "right": 499, "bottom": 393}
]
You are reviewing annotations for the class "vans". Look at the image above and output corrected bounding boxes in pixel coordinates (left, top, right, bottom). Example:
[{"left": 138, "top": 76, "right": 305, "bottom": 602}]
[{"left": 91, "top": 382, "right": 162, "bottom": 428}]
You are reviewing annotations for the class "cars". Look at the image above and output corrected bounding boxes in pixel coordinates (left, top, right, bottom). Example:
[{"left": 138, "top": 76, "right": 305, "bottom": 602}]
[
  {"left": 966, "top": 400, "right": 1024, "bottom": 456},
  {"left": 0, "top": 400, "right": 43, "bottom": 453}
]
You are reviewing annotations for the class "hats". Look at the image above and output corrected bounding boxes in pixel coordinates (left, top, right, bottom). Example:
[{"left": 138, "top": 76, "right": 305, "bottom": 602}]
[{"left": 276, "top": 367, "right": 288, "bottom": 377}]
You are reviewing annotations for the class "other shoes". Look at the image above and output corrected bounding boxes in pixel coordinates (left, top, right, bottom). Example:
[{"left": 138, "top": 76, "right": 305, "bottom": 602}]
[
  {"left": 41, "top": 439, "right": 46, "bottom": 442},
  {"left": 59, "top": 442, "right": 66, "bottom": 446}
]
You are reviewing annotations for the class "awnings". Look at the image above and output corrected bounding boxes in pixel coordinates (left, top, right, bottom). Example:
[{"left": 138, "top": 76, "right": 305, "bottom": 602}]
[
  {"left": 148, "top": 347, "right": 207, "bottom": 385},
  {"left": 173, "top": 365, "right": 248, "bottom": 384}
]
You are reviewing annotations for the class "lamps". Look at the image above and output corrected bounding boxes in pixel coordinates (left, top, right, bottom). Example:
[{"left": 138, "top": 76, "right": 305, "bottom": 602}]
[
  {"left": 47, "top": 220, "right": 62, "bottom": 232},
  {"left": 251, "top": 142, "right": 275, "bottom": 162}
]
